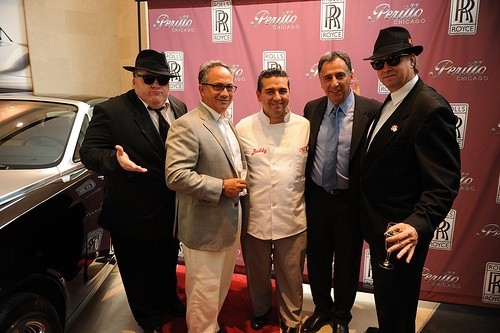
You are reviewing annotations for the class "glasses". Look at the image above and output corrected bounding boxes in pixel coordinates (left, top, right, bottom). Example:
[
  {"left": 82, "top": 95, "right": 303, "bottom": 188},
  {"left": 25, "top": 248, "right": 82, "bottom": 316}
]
[
  {"left": 201, "top": 82, "right": 237, "bottom": 93},
  {"left": 370, "top": 52, "right": 410, "bottom": 70},
  {"left": 135, "top": 73, "right": 170, "bottom": 86}
]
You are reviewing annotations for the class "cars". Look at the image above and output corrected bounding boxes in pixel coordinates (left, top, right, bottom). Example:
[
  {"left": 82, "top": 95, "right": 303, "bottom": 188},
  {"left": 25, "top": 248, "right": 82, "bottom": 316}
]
[{"left": 0, "top": 91, "right": 118, "bottom": 333}]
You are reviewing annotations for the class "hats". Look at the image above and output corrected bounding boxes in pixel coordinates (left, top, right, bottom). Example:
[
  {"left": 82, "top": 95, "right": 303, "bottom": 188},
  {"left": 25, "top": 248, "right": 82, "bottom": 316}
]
[
  {"left": 123, "top": 50, "right": 180, "bottom": 78},
  {"left": 363, "top": 26, "right": 424, "bottom": 60}
]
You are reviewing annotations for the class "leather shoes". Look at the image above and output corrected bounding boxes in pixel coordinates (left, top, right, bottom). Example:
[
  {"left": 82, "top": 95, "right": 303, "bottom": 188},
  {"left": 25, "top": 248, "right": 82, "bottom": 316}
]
[
  {"left": 301, "top": 312, "right": 331, "bottom": 333},
  {"left": 144, "top": 321, "right": 161, "bottom": 333},
  {"left": 252, "top": 305, "right": 272, "bottom": 329},
  {"left": 332, "top": 318, "right": 349, "bottom": 333},
  {"left": 163, "top": 298, "right": 186, "bottom": 317},
  {"left": 280, "top": 321, "right": 296, "bottom": 333}
]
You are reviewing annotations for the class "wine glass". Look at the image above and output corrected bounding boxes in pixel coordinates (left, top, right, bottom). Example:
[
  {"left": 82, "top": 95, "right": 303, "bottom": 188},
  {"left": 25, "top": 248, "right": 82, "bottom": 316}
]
[
  {"left": 237, "top": 160, "right": 248, "bottom": 196},
  {"left": 379, "top": 222, "right": 401, "bottom": 269}
]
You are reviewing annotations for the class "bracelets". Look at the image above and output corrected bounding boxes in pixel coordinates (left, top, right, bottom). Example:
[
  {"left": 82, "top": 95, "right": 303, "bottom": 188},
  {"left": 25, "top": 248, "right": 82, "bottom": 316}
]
[{"left": 222, "top": 187, "right": 225, "bottom": 192}]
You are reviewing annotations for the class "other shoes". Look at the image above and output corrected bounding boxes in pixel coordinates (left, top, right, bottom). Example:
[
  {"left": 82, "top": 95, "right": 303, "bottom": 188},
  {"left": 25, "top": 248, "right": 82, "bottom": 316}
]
[{"left": 366, "top": 327, "right": 382, "bottom": 333}]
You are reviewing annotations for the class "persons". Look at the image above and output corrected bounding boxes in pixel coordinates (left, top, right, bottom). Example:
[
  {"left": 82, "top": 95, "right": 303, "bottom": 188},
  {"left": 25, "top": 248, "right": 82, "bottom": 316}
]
[
  {"left": 300, "top": 51, "right": 382, "bottom": 333},
  {"left": 78, "top": 49, "right": 188, "bottom": 333},
  {"left": 360, "top": 26, "right": 461, "bottom": 333},
  {"left": 234, "top": 69, "right": 310, "bottom": 333},
  {"left": 165, "top": 62, "right": 248, "bottom": 333}
]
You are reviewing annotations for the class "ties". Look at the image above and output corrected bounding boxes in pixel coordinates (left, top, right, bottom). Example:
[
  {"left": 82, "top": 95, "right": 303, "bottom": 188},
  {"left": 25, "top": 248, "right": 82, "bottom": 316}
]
[
  {"left": 360, "top": 92, "right": 392, "bottom": 160},
  {"left": 321, "top": 105, "right": 341, "bottom": 193}
]
